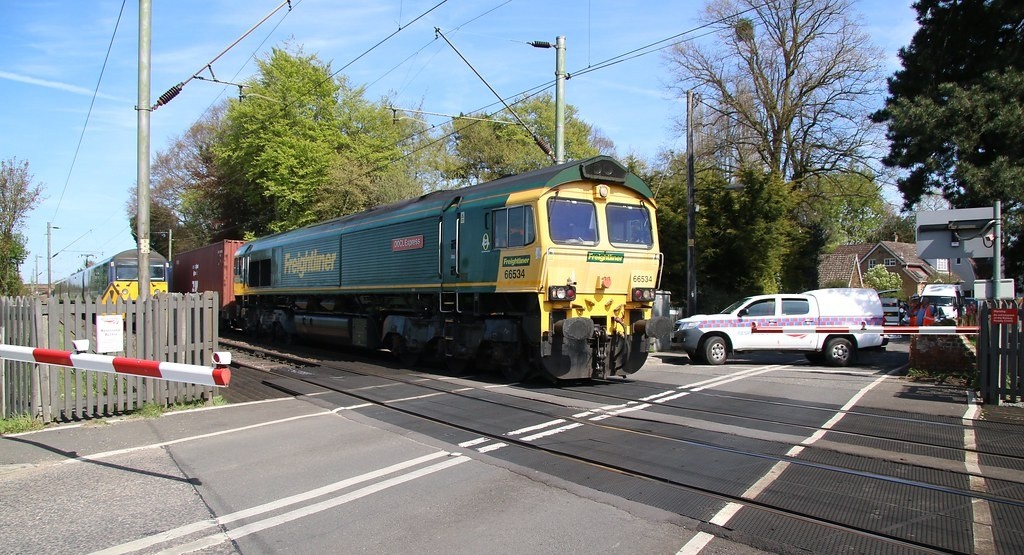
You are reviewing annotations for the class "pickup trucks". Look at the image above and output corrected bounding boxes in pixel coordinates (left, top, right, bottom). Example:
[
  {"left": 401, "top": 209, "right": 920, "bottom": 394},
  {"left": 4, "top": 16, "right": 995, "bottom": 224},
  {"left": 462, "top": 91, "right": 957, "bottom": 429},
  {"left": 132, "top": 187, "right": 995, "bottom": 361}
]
[{"left": 674, "top": 288, "right": 887, "bottom": 367}]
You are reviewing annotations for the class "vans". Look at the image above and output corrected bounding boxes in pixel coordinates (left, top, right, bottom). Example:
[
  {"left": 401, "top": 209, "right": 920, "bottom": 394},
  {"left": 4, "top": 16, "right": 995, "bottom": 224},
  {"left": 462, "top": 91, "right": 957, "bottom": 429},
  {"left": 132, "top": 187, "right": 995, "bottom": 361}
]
[{"left": 921, "top": 284, "right": 965, "bottom": 326}]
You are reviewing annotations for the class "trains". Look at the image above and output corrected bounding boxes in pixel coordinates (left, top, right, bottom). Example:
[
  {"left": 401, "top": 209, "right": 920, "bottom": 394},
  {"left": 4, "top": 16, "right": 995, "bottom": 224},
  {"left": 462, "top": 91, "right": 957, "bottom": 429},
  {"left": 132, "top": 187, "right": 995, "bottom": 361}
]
[
  {"left": 174, "top": 155, "right": 672, "bottom": 383},
  {"left": 53, "top": 247, "right": 172, "bottom": 323}
]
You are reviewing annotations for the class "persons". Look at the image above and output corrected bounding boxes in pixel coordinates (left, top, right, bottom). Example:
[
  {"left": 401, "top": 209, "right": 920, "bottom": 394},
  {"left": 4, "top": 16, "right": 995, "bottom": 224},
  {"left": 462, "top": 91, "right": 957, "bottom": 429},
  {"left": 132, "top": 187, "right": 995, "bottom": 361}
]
[
  {"left": 899, "top": 293, "right": 938, "bottom": 326},
  {"left": 965, "top": 301, "right": 976, "bottom": 325},
  {"left": 509, "top": 217, "right": 526, "bottom": 246}
]
